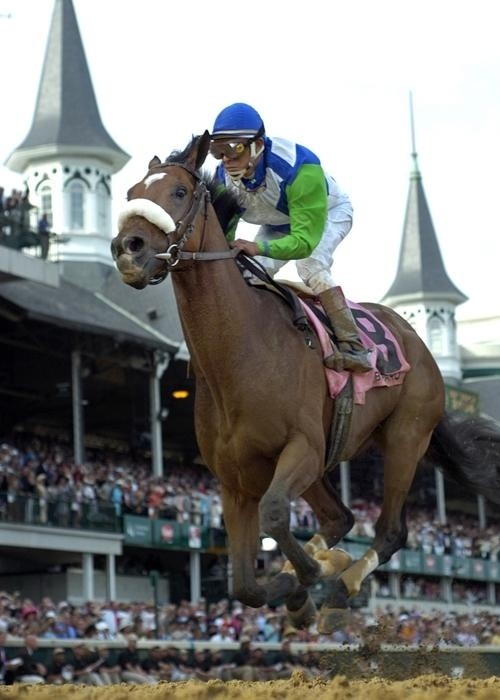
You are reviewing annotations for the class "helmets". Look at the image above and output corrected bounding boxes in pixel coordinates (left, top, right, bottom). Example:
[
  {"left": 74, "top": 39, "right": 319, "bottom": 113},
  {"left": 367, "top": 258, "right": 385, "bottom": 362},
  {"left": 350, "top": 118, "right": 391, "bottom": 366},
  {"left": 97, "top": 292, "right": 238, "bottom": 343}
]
[{"left": 209, "top": 103, "right": 268, "bottom": 142}]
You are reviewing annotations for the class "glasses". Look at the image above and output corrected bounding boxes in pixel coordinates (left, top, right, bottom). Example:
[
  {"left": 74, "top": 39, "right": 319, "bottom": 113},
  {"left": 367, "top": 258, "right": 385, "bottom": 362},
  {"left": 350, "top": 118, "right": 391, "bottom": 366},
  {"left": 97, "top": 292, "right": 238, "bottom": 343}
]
[{"left": 208, "top": 130, "right": 261, "bottom": 159}]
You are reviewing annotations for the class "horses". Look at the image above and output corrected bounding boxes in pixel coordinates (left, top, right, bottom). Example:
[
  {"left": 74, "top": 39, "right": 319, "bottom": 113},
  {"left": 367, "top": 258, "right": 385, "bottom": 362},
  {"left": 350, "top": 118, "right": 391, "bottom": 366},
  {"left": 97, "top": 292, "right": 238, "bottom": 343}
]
[{"left": 110, "top": 129, "right": 500, "bottom": 634}]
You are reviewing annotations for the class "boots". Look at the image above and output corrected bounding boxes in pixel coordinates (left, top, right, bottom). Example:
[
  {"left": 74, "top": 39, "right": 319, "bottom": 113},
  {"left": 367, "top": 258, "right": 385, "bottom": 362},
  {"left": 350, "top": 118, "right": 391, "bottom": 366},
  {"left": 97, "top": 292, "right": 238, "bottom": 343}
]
[{"left": 316, "top": 286, "right": 373, "bottom": 374}]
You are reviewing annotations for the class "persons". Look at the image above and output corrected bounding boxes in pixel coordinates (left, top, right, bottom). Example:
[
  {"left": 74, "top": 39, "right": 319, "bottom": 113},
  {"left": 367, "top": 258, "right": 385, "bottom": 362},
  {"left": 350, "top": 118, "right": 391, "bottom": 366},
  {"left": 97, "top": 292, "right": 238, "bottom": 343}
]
[
  {"left": 0, "top": 178, "right": 54, "bottom": 261},
  {"left": 0, "top": 435, "right": 500, "bottom": 682},
  {"left": 204, "top": 102, "right": 375, "bottom": 376}
]
